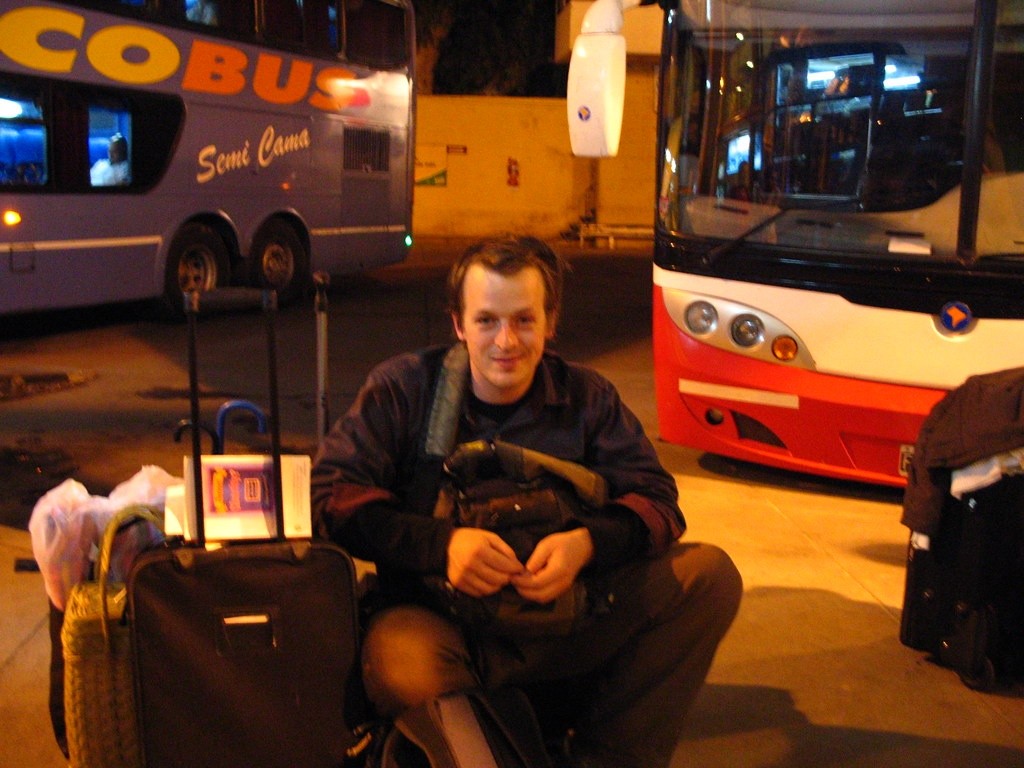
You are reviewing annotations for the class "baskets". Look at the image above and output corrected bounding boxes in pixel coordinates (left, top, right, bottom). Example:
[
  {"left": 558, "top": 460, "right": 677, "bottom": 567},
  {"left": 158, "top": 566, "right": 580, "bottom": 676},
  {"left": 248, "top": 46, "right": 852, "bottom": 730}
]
[{"left": 61, "top": 505, "right": 166, "bottom": 768}]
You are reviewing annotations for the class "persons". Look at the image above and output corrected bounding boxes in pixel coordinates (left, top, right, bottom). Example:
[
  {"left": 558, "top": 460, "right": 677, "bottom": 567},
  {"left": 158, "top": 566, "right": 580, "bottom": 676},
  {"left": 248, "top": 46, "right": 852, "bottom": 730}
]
[
  {"left": 737, "top": 161, "right": 787, "bottom": 210},
  {"left": 90, "top": 132, "right": 132, "bottom": 188},
  {"left": 309, "top": 235, "right": 742, "bottom": 768},
  {"left": 10, "top": 164, "right": 44, "bottom": 184}
]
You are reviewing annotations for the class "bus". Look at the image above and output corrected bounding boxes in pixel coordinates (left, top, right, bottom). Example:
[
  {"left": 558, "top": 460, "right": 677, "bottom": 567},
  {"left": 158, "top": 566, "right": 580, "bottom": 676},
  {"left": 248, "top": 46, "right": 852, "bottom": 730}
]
[
  {"left": 565, "top": 0, "right": 1023, "bottom": 490},
  {"left": 1, "top": 1, "right": 420, "bottom": 329}
]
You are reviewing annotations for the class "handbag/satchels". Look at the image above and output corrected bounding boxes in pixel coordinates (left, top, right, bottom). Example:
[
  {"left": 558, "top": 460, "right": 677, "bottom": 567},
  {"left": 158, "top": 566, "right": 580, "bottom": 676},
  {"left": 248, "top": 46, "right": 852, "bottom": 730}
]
[{"left": 433, "top": 448, "right": 595, "bottom": 640}]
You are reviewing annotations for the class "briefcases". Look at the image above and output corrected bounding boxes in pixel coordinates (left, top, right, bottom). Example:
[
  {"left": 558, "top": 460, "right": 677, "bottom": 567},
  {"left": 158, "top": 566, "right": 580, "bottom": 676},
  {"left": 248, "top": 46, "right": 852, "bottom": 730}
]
[{"left": 901, "top": 476, "right": 1023, "bottom": 691}]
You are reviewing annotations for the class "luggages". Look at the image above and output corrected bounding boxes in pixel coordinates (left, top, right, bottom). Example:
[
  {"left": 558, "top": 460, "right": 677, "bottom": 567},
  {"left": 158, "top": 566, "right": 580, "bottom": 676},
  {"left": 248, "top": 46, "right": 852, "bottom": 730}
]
[{"left": 125, "top": 281, "right": 376, "bottom": 768}]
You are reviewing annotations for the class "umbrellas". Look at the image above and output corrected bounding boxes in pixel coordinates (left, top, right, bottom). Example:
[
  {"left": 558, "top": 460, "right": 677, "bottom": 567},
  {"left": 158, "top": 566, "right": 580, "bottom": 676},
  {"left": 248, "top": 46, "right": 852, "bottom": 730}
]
[{"left": 173, "top": 400, "right": 266, "bottom": 455}]
